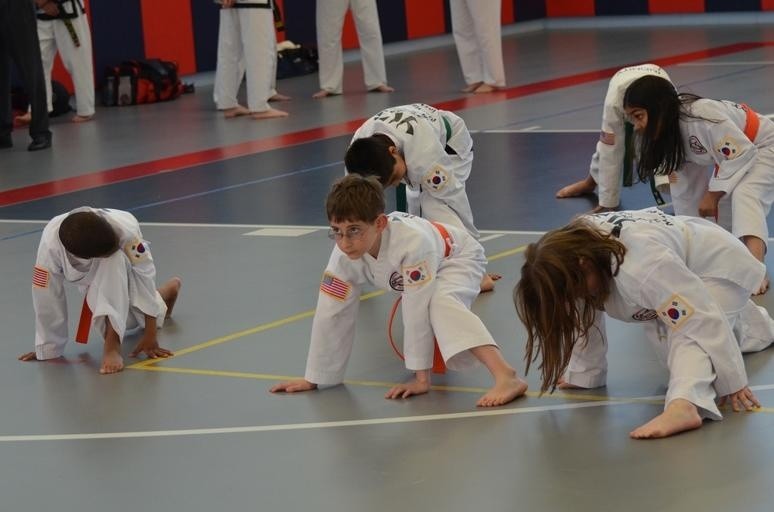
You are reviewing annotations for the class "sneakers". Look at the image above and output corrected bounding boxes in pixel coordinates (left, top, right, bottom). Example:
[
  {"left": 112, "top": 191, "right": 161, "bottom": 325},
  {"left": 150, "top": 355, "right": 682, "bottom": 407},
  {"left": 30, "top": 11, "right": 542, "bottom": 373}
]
[
  {"left": 27, "top": 135, "right": 52, "bottom": 151},
  {"left": 0, "top": 140, "right": 13, "bottom": 149}
]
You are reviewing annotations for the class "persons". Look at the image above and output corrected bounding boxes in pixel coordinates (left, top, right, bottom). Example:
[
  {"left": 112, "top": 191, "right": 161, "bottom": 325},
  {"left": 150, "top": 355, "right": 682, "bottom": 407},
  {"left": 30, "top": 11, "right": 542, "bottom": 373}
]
[
  {"left": 342, "top": 101, "right": 503, "bottom": 296},
  {"left": 11, "top": 0, "right": 98, "bottom": 128},
  {"left": 449, "top": 0, "right": 507, "bottom": 94},
  {"left": 310, "top": 0, "right": 396, "bottom": 99},
  {"left": 267, "top": 174, "right": 529, "bottom": 408},
  {"left": 212, "top": 0, "right": 289, "bottom": 119},
  {"left": 621, "top": 75, "right": 774, "bottom": 295},
  {"left": 512, "top": 206, "right": 774, "bottom": 440},
  {"left": 235, "top": 27, "right": 292, "bottom": 103},
  {"left": 18, "top": 205, "right": 183, "bottom": 376},
  {"left": 1, "top": 0, "right": 53, "bottom": 153},
  {"left": 558, "top": 62, "right": 683, "bottom": 215}
]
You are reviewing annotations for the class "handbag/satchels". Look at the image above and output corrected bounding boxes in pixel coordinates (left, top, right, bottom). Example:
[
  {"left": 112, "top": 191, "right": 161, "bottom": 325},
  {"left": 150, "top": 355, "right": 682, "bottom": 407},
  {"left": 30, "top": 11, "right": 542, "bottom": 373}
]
[
  {"left": 277, "top": 44, "right": 306, "bottom": 79},
  {"left": 113, "top": 59, "right": 183, "bottom": 105}
]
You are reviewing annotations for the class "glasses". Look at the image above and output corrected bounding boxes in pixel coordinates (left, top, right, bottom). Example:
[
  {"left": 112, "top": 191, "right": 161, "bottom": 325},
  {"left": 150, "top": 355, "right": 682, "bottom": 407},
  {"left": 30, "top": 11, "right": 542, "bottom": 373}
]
[{"left": 328, "top": 225, "right": 370, "bottom": 240}]
